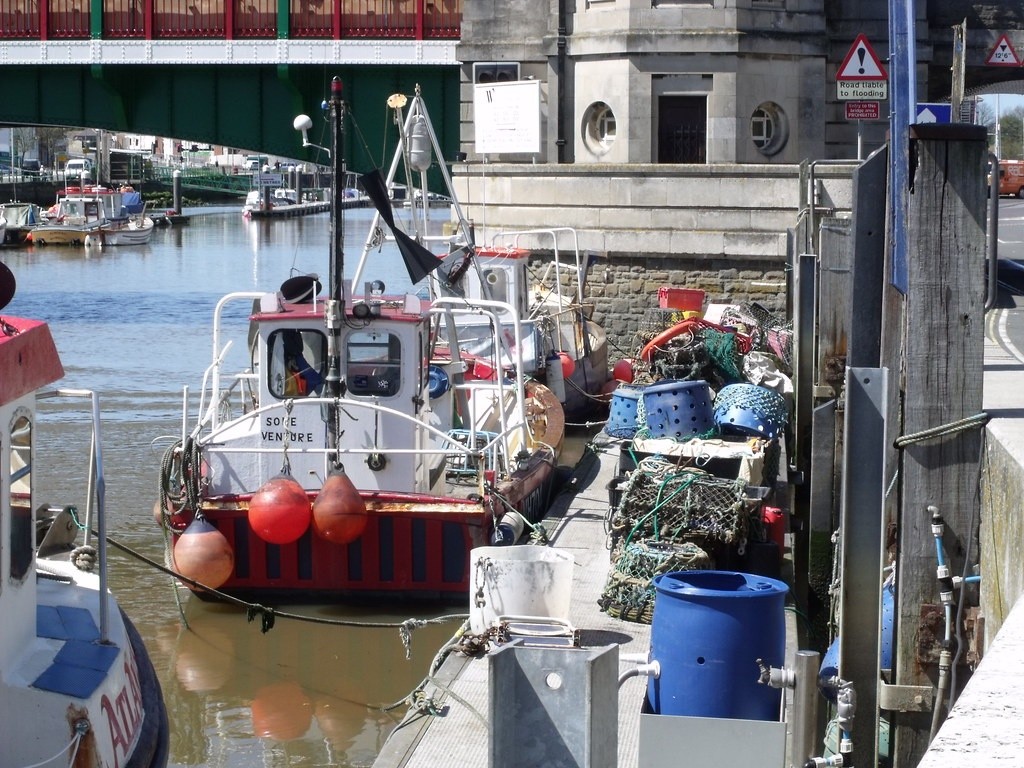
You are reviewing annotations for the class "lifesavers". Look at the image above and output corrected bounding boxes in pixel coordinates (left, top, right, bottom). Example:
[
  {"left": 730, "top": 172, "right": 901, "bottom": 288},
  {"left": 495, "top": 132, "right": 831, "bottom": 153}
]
[{"left": 641, "top": 321, "right": 700, "bottom": 362}]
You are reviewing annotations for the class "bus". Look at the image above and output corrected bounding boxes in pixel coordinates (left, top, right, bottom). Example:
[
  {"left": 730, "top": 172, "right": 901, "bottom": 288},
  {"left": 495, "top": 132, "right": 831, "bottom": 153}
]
[{"left": 247, "top": 157, "right": 268, "bottom": 170}]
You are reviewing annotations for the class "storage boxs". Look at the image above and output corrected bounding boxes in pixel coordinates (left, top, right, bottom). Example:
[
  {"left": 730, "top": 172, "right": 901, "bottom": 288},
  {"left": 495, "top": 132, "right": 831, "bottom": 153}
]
[
  {"left": 656, "top": 286, "right": 705, "bottom": 313},
  {"left": 605, "top": 441, "right": 770, "bottom": 542}
]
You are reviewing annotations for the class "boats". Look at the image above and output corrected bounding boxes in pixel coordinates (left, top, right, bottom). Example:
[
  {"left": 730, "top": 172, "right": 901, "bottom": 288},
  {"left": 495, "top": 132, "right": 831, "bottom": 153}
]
[
  {"left": 164, "top": 74, "right": 564, "bottom": 599},
  {"left": 105, "top": 217, "right": 154, "bottom": 246},
  {"left": 29, "top": 190, "right": 129, "bottom": 249},
  {"left": 240, "top": 188, "right": 307, "bottom": 211},
  {"left": 0, "top": 314, "right": 172, "bottom": 767},
  {"left": 0, "top": 202, "right": 46, "bottom": 246}
]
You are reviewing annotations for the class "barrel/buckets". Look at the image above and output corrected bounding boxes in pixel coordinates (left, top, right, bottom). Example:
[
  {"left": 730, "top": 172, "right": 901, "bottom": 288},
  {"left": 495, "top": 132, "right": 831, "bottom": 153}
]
[
  {"left": 469, "top": 545, "right": 576, "bottom": 636},
  {"left": 645, "top": 571, "right": 789, "bottom": 723}
]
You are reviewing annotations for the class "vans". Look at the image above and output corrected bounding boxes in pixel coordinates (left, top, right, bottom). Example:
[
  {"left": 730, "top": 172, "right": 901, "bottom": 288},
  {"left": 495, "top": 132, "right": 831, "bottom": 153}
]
[
  {"left": 272, "top": 162, "right": 295, "bottom": 173},
  {"left": 22, "top": 159, "right": 42, "bottom": 176},
  {"left": 64, "top": 160, "right": 91, "bottom": 180},
  {"left": 243, "top": 160, "right": 259, "bottom": 170}
]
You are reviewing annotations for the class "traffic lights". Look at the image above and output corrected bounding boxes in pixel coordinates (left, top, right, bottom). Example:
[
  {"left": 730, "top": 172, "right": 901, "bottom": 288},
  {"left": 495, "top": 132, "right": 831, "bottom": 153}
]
[{"left": 177, "top": 143, "right": 181, "bottom": 151}]
